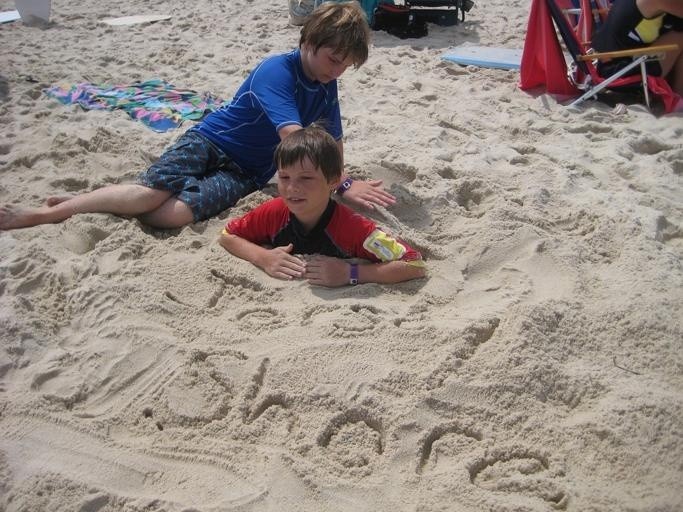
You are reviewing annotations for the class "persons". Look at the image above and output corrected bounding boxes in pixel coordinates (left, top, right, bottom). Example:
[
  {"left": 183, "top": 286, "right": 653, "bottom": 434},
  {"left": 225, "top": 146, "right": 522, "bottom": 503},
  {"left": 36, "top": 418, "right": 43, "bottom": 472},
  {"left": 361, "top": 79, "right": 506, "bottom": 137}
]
[
  {"left": 596, "top": 0, "right": 682, "bottom": 107},
  {"left": 217, "top": 125, "right": 427, "bottom": 285},
  {"left": 0, "top": 0, "right": 399, "bottom": 233}
]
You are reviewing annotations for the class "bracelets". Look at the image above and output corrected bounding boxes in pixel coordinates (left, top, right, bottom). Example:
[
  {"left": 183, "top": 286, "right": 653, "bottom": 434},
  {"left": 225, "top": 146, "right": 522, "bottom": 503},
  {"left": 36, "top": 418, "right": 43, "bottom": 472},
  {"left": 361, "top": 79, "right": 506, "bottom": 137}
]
[
  {"left": 348, "top": 263, "right": 360, "bottom": 286},
  {"left": 334, "top": 177, "right": 353, "bottom": 195}
]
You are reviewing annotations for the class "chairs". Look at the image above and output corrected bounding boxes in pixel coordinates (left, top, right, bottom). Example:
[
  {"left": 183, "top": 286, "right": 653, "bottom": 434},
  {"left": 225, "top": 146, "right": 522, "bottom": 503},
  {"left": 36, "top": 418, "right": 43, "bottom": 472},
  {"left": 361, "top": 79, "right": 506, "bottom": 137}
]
[
  {"left": 595, "top": 0, "right": 613, "bottom": 23},
  {"left": 546, "top": 0, "right": 679, "bottom": 112}
]
